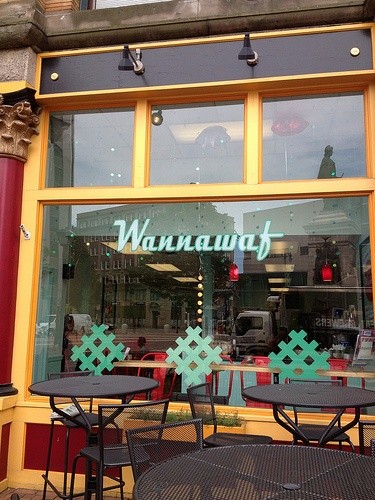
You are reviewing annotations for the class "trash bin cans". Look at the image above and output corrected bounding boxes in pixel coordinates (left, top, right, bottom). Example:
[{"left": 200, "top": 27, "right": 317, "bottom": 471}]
[
  {"left": 122, "top": 324, "right": 128, "bottom": 334},
  {"left": 164, "top": 325, "right": 169, "bottom": 333}
]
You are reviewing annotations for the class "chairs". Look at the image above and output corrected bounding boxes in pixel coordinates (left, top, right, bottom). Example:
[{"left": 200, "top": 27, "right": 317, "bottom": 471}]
[
  {"left": 206, "top": 355, "right": 234, "bottom": 397},
  {"left": 125, "top": 417, "right": 203, "bottom": 483},
  {"left": 186, "top": 382, "right": 273, "bottom": 447},
  {"left": 42, "top": 369, "right": 127, "bottom": 500},
  {"left": 288, "top": 378, "right": 356, "bottom": 453},
  {"left": 239, "top": 353, "right": 285, "bottom": 409},
  {"left": 126, "top": 352, "right": 176, "bottom": 401},
  {"left": 320, "top": 359, "right": 365, "bottom": 414},
  {"left": 359, "top": 419, "right": 375, "bottom": 457},
  {"left": 69, "top": 398, "right": 169, "bottom": 500}
]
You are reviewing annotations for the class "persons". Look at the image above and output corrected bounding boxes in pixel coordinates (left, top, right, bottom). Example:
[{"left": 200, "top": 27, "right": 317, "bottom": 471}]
[
  {"left": 61, "top": 328, "right": 69, "bottom": 371},
  {"left": 318, "top": 145, "right": 337, "bottom": 178},
  {"left": 135, "top": 337, "right": 149, "bottom": 360}
]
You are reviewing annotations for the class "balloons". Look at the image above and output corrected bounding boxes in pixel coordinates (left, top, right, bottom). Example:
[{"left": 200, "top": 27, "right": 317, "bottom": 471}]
[
  {"left": 196, "top": 127, "right": 231, "bottom": 149},
  {"left": 271, "top": 118, "right": 308, "bottom": 135}
]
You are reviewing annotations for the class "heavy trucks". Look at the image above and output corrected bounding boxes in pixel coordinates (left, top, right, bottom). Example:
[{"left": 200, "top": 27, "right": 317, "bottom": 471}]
[{"left": 229, "top": 283, "right": 373, "bottom": 364}]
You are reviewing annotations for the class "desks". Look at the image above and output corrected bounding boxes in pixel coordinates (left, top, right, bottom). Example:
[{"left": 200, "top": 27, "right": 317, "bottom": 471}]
[
  {"left": 28, "top": 375, "right": 160, "bottom": 500},
  {"left": 242, "top": 384, "right": 375, "bottom": 447},
  {"left": 112, "top": 360, "right": 375, "bottom": 413},
  {"left": 132, "top": 444, "right": 375, "bottom": 500}
]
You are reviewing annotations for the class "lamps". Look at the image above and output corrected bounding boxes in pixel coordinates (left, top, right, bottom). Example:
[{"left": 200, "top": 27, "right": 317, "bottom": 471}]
[
  {"left": 229, "top": 251, "right": 239, "bottom": 281},
  {"left": 152, "top": 110, "right": 163, "bottom": 125},
  {"left": 321, "top": 237, "right": 333, "bottom": 282},
  {"left": 238, "top": 32, "right": 259, "bottom": 65},
  {"left": 271, "top": 110, "right": 309, "bottom": 136},
  {"left": 118, "top": 44, "right": 145, "bottom": 75}
]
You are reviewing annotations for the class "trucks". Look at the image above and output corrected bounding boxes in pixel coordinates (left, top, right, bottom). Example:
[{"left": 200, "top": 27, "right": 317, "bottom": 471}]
[{"left": 37, "top": 312, "right": 93, "bottom": 337}]
